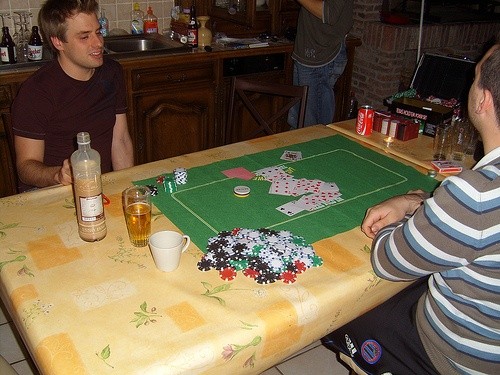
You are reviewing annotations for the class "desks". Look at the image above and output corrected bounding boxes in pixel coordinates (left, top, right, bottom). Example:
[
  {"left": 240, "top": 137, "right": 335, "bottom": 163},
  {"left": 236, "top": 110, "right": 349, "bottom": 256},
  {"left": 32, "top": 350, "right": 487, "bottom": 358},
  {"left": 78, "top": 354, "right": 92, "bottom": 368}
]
[{"left": 0, "top": 118, "right": 475, "bottom": 375}]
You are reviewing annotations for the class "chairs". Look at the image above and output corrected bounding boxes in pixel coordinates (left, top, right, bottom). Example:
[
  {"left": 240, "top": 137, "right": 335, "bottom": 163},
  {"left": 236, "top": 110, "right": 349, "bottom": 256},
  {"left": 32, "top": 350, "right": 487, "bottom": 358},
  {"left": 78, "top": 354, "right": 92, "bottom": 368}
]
[{"left": 229, "top": 76, "right": 309, "bottom": 144}]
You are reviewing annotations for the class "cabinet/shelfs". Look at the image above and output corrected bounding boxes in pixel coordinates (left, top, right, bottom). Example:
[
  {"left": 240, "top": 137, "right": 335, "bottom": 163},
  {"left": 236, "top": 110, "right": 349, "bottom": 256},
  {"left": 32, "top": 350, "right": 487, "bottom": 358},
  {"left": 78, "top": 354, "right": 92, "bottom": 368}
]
[{"left": 0, "top": 0, "right": 362, "bottom": 199}]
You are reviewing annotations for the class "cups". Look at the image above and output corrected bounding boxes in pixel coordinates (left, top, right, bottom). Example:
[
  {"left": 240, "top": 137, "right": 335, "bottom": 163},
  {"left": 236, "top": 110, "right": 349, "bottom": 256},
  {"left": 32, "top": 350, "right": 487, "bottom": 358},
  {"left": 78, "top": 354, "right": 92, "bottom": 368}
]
[
  {"left": 433, "top": 126, "right": 448, "bottom": 161},
  {"left": 466, "top": 122, "right": 478, "bottom": 158},
  {"left": 122, "top": 186, "right": 152, "bottom": 248},
  {"left": 444, "top": 127, "right": 455, "bottom": 162},
  {"left": 148, "top": 230, "right": 190, "bottom": 273},
  {"left": 453, "top": 120, "right": 468, "bottom": 161}
]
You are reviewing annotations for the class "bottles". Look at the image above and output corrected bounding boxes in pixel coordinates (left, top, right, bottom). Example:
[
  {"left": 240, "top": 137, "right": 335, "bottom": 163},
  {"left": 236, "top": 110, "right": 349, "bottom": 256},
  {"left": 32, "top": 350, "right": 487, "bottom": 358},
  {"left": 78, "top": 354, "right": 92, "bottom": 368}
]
[
  {"left": 197, "top": 15, "right": 213, "bottom": 48},
  {"left": 27, "top": 26, "right": 43, "bottom": 62},
  {"left": 70, "top": 132, "right": 107, "bottom": 242},
  {"left": 169, "top": 3, "right": 198, "bottom": 47},
  {"left": 144, "top": 6, "right": 158, "bottom": 35},
  {"left": 130, "top": 3, "right": 144, "bottom": 35},
  {"left": 343, "top": 88, "right": 356, "bottom": 120},
  {"left": 99, "top": 11, "right": 109, "bottom": 38},
  {"left": 0, "top": 26, "right": 17, "bottom": 64}
]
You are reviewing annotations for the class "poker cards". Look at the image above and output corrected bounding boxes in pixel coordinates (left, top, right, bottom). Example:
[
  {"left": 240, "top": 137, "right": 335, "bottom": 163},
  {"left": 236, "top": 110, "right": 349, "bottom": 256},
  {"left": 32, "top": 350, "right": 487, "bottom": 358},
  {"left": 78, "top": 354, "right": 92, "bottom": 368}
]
[
  {"left": 220, "top": 166, "right": 258, "bottom": 180},
  {"left": 280, "top": 151, "right": 302, "bottom": 162},
  {"left": 256, "top": 166, "right": 342, "bottom": 217}
]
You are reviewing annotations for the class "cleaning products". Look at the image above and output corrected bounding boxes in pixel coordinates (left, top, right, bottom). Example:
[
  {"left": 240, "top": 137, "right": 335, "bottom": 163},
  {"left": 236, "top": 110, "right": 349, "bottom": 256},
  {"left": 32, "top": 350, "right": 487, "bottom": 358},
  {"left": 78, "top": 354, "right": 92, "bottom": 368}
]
[
  {"left": 143, "top": 6, "right": 158, "bottom": 34},
  {"left": 97, "top": 8, "right": 109, "bottom": 38},
  {"left": 130, "top": 2, "right": 144, "bottom": 35}
]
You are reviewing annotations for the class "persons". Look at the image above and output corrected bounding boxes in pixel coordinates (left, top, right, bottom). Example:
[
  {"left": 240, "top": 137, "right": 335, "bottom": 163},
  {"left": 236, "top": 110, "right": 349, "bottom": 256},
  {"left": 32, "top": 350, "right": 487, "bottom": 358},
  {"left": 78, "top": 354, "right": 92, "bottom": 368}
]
[
  {"left": 286, "top": 0, "right": 354, "bottom": 129},
  {"left": 326, "top": 43, "right": 500, "bottom": 375},
  {"left": 12, "top": 0, "right": 135, "bottom": 191}
]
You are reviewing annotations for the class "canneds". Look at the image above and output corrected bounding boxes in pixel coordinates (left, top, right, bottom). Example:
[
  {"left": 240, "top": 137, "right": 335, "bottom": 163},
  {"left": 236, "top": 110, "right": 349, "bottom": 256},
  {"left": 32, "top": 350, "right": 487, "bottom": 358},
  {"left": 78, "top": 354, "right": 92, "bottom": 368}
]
[{"left": 355, "top": 105, "right": 374, "bottom": 136}]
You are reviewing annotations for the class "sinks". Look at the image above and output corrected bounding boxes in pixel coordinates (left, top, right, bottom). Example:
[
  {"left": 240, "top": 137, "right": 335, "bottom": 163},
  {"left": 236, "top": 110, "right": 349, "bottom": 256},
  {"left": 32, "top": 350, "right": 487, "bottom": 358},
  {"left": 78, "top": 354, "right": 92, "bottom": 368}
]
[
  {"left": 103, "top": 49, "right": 109, "bottom": 55},
  {"left": 103, "top": 33, "right": 192, "bottom": 53}
]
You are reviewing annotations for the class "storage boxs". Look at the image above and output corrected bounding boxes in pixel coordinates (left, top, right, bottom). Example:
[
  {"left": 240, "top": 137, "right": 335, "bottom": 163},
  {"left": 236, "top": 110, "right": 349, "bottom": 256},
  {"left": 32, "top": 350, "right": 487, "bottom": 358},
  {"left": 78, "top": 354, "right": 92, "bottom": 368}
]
[{"left": 383, "top": 93, "right": 454, "bottom": 138}]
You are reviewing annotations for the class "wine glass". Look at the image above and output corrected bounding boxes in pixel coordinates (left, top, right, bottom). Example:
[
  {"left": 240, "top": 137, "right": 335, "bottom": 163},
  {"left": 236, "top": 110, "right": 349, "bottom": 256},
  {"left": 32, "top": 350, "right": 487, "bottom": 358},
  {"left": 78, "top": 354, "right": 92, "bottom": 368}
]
[{"left": 0, "top": 10, "right": 32, "bottom": 63}]
